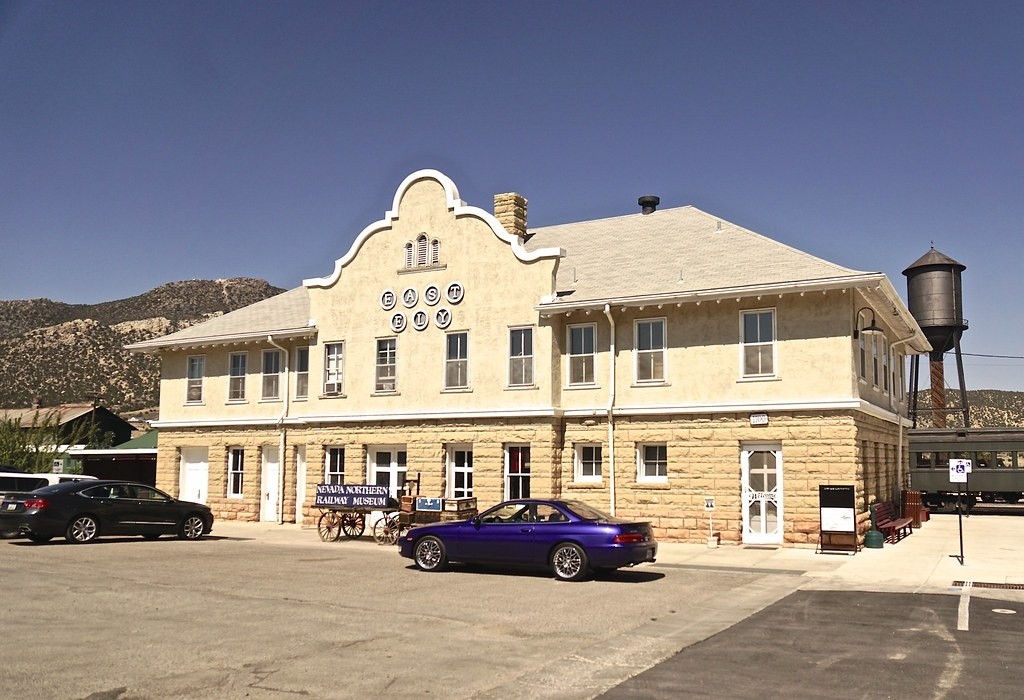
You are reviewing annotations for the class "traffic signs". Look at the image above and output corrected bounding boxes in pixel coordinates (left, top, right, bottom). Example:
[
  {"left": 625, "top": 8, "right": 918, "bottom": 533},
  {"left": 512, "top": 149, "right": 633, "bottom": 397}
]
[
  {"left": 949, "top": 458, "right": 968, "bottom": 483},
  {"left": 966, "top": 460, "right": 972, "bottom": 474}
]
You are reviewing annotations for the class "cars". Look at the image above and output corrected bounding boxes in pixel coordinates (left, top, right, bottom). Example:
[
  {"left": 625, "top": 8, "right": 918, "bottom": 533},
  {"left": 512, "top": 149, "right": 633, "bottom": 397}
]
[
  {"left": 397, "top": 497, "right": 658, "bottom": 581},
  {"left": 0, "top": 478, "right": 214, "bottom": 544}
]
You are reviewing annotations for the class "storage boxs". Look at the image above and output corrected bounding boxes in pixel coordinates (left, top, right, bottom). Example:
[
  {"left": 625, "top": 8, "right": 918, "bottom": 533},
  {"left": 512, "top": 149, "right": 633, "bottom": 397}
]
[
  {"left": 416, "top": 497, "right": 445, "bottom": 512},
  {"left": 402, "top": 495, "right": 427, "bottom": 504},
  {"left": 445, "top": 497, "right": 477, "bottom": 511},
  {"left": 401, "top": 504, "right": 415, "bottom": 512}
]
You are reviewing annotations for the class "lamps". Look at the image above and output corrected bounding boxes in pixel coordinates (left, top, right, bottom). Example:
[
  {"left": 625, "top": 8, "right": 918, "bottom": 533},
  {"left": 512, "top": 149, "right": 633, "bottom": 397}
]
[
  {"left": 540, "top": 315, "right": 547, "bottom": 319},
  {"left": 757, "top": 294, "right": 763, "bottom": 300},
  {"left": 697, "top": 300, "right": 702, "bottom": 306},
  {"left": 800, "top": 289, "right": 805, "bottom": 297},
  {"left": 716, "top": 298, "right": 721, "bottom": 304},
  {"left": 565, "top": 312, "right": 572, "bottom": 317},
  {"left": 676, "top": 302, "right": 683, "bottom": 308},
  {"left": 547, "top": 314, "right": 553, "bottom": 318},
  {"left": 736, "top": 296, "right": 742, "bottom": 302},
  {"left": 875, "top": 285, "right": 880, "bottom": 291},
  {"left": 854, "top": 307, "right": 885, "bottom": 338},
  {"left": 866, "top": 286, "right": 872, "bottom": 292},
  {"left": 841, "top": 288, "right": 846, "bottom": 294},
  {"left": 778, "top": 291, "right": 784, "bottom": 298},
  {"left": 822, "top": 288, "right": 827, "bottom": 295},
  {"left": 639, "top": 305, "right": 645, "bottom": 311},
  {"left": 129, "top": 336, "right": 315, "bottom": 358},
  {"left": 658, "top": 304, "right": 663, "bottom": 309},
  {"left": 586, "top": 310, "right": 592, "bottom": 315},
  {"left": 620, "top": 307, "right": 627, "bottom": 313}
]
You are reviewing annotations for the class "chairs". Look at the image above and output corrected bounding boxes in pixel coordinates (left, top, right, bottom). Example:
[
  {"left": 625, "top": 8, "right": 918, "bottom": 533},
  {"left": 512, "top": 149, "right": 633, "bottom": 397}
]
[{"left": 116, "top": 487, "right": 128, "bottom": 498}]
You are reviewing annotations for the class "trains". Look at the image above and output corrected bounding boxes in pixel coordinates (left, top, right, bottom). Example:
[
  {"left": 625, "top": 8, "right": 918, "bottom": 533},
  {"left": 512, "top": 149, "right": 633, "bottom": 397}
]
[{"left": 908, "top": 427, "right": 1024, "bottom": 511}]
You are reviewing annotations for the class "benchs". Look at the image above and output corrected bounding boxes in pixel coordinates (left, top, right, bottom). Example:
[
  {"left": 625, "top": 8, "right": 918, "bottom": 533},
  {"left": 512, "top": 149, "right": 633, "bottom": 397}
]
[
  {"left": 871, "top": 501, "right": 913, "bottom": 543},
  {"left": 922, "top": 508, "right": 930, "bottom": 522}
]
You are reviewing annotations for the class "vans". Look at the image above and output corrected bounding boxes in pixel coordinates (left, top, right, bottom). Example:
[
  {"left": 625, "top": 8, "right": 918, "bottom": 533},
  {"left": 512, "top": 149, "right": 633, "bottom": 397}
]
[{"left": 0, "top": 472, "right": 100, "bottom": 539}]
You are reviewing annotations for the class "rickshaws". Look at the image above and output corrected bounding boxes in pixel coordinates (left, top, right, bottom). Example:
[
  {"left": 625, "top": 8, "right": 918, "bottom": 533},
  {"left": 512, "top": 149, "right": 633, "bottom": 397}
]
[{"left": 311, "top": 472, "right": 421, "bottom": 546}]
